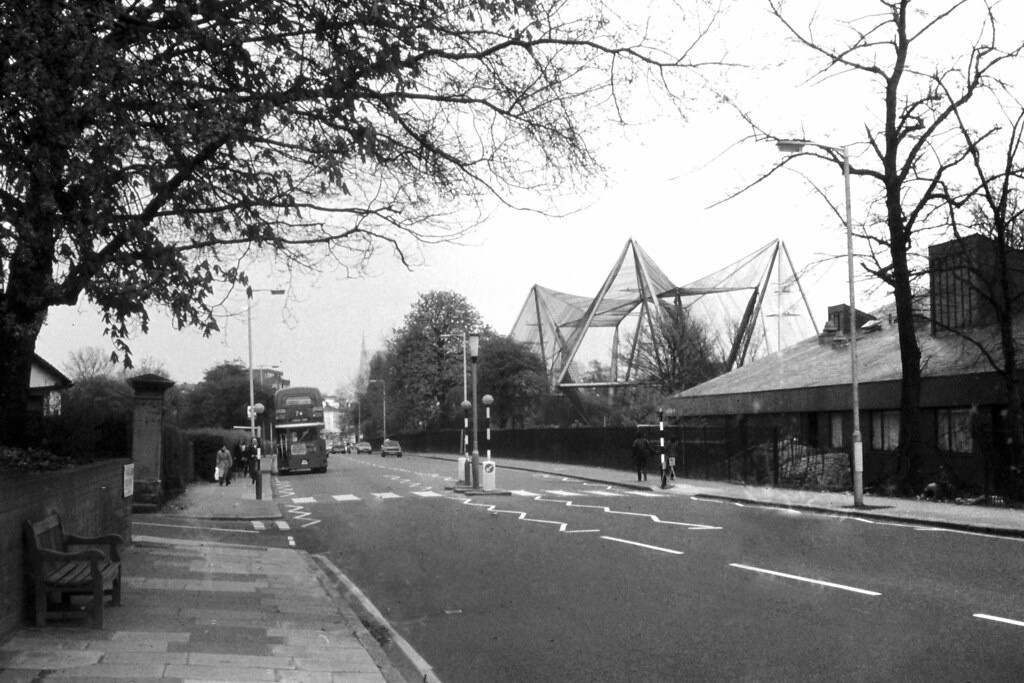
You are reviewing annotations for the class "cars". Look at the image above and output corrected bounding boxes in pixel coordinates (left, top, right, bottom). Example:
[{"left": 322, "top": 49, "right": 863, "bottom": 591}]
[
  {"left": 355, "top": 441, "right": 373, "bottom": 455},
  {"left": 325, "top": 438, "right": 356, "bottom": 455},
  {"left": 381, "top": 439, "right": 403, "bottom": 458}
]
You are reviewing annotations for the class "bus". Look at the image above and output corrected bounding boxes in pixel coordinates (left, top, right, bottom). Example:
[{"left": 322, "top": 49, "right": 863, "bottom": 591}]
[{"left": 269, "top": 386, "right": 329, "bottom": 476}]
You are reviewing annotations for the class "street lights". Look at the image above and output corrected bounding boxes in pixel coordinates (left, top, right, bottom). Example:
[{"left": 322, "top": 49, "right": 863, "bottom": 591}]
[
  {"left": 440, "top": 332, "right": 471, "bottom": 487},
  {"left": 369, "top": 379, "right": 386, "bottom": 440},
  {"left": 776, "top": 137, "right": 865, "bottom": 510},
  {"left": 246, "top": 284, "right": 285, "bottom": 439},
  {"left": 351, "top": 400, "right": 361, "bottom": 442}
]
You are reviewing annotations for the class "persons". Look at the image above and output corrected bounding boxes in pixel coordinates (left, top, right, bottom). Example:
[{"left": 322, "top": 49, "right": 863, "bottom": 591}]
[
  {"left": 248, "top": 438, "right": 260, "bottom": 484},
  {"left": 216, "top": 443, "right": 233, "bottom": 487},
  {"left": 665, "top": 434, "right": 679, "bottom": 480},
  {"left": 233, "top": 439, "right": 248, "bottom": 477},
  {"left": 632, "top": 431, "right": 659, "bottom": 482}
]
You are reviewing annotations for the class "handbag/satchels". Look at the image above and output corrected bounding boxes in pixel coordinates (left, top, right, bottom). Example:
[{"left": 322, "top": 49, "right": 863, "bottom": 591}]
[
  {"left": 242, "top": 455, "right": 247, "bottom": 461},
  {"left": 214, "top": 467, "right": 219, "bottom": 480},
  {"left": 669, "top": 457, "right": 675, "bottom": 466}
]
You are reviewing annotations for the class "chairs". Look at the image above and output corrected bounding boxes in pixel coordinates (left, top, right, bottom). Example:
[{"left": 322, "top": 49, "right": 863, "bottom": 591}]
[{"left": 24, "top": 507, "right": 122, "bottom": 630}]
